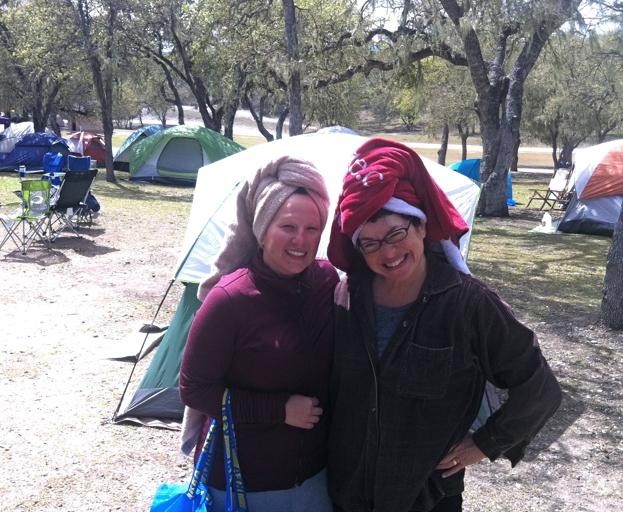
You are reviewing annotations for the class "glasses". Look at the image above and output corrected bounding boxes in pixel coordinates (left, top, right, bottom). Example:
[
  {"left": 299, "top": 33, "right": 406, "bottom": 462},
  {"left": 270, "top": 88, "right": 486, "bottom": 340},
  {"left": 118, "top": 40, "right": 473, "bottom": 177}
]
[{"left": 355, "top": 218, "right": 414, "bottom": 256}]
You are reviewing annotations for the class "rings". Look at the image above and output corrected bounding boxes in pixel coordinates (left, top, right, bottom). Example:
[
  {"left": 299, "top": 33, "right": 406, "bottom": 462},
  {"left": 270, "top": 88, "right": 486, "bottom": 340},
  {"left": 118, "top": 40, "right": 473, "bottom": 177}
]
[{"left": 452, "top": 458, "right": 457, "bottom": 466}]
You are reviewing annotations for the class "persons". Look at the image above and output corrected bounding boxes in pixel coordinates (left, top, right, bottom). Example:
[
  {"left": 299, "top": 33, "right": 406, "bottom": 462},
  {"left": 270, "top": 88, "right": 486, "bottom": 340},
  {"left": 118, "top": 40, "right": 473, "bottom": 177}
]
[
  {"left": 178, "top": 154, "right": 354, "bottom": 512},
  {"left": 327, "top": 136, "right": 565, "bottom": 511}
]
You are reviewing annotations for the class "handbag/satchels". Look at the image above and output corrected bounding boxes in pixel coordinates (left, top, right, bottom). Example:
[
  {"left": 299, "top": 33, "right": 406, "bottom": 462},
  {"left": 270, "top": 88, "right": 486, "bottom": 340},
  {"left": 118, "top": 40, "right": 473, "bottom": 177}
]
[{"left": 149, "top": 384, "right": 251, "bottom": 512}]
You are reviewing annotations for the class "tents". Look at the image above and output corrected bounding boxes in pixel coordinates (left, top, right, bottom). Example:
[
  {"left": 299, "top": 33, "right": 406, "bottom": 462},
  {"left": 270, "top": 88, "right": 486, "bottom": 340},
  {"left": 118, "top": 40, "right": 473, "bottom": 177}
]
[
  {"left": 444, "top": 156, "right": 521, "bottom": 207},
  {"left": 551, "top": 141, "right": 622, "bottom": 240},
  {"left": 311, "top": 123, "right": 357, "bottom": 135},
  {"left": 0, "top": 112, "right": 248, "bottom": 187},
  {"left": 113, "top": 131, "right": 499, "bottom": 440},
  {"left": 541, "top": 135, "right": 623, "bottom": 206}
]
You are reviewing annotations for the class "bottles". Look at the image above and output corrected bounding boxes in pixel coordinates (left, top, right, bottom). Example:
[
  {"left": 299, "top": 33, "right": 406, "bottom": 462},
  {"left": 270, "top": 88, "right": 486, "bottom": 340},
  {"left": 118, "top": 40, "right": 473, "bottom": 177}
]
[{"left": 541, "top": 212, "right": 552, "bottom": 231}]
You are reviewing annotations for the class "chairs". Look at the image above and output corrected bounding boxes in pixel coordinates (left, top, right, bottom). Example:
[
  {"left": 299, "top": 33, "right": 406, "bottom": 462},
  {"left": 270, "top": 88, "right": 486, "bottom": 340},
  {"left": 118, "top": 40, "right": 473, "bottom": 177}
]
[
  {"left": 0, "top": 150, "right": 101, "bottom": 261},
  {"left": 524, "top": 165, "right": 572, "bottom": 214}
]
